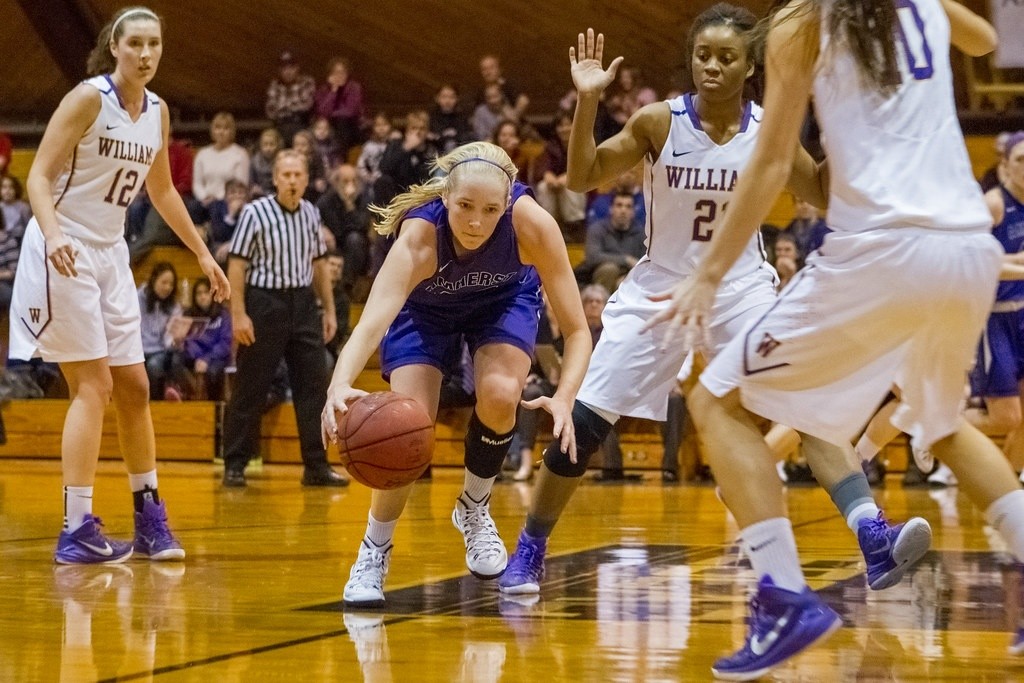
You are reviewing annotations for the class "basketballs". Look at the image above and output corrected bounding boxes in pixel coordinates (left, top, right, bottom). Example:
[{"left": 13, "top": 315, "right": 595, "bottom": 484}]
[{"left": 335, "top": 391, "right": 436, "bottom": 491}]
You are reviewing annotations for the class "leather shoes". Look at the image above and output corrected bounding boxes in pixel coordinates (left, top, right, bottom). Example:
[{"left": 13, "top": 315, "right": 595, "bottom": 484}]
[
  {"left": 223, "top": 469, "right": 245, "bottom": 486},
  {"left": 302, "top": 468, "right": 350, "bottom": 486}
]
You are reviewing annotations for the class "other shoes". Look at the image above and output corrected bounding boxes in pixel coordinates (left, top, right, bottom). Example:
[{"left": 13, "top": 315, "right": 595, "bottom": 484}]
[
  {"left": 513, "top": 457, "right": 533, "bottom": 481},
  {"left": 244, "top": 457, "right": 263, "bottom": 476},
  {"left": 716, "top": 487, "right": 722, "bottom": 502},
  {"left": 861, "top": 460, "right": 881, "bottom": 483},
  {"left": 593, "top": 470, "right": 676, "bottom": 481},
  {"left": 904, "top": 464, "right": 921, "bottom": 484},
  {"left": 777, "top": 460, "right": 816, "bottom": 482},
  {"left": 165, "top": 387, "right": 182, "bottom": 401}
]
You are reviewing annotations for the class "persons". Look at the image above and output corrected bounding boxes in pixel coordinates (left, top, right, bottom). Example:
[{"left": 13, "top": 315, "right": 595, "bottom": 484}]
[
  {"left": 0, "top": 47, "right": 1023, "bottom": 485},
  {"left": 313, "top": 141, "right": 594, "bottom": 601},
  {"left": 635, "top": 2, "right": 1023, "bottom": 679},
  {"left": 504, "top": 3, "right": 933, "bottom": 592},
  {"left": 6, "top": 5, "right": 235, "bottom": 564}
]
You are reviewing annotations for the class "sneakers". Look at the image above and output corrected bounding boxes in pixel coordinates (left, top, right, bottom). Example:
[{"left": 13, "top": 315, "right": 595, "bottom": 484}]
[
  {"left": 54, "top": 515, "right": 133, "bottom": 565},
  {"left": 1007, "top": 628, "right": 1024, "bottom": 654},
  {"left": 912, "top": 446, "right": 934, "bottom": 473},
  {"left": 452, "top": 497, "right": 508, "bottom": 580},
  {"left": 343, "top": 540, "right": 394, "bottom": 607},
  {"left": 131, "top": 498, "right": 186, "bottom": 562},
  {"left": 711, "top": 573, "right": 843, "bottom": 683},
  {"left": 857, "top": 510, "right": 932, "bottom": 590},
  {"left": 498, "top": 528, "right": 547, "bottom": 594},
  {"left": 927, "top": 464, "right": 957, "bottom": 486}
]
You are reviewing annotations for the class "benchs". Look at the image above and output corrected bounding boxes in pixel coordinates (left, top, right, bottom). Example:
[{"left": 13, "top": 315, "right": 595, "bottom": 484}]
[{"left": 0, "top": 136, "right": 1015, "bottom": 468}]
